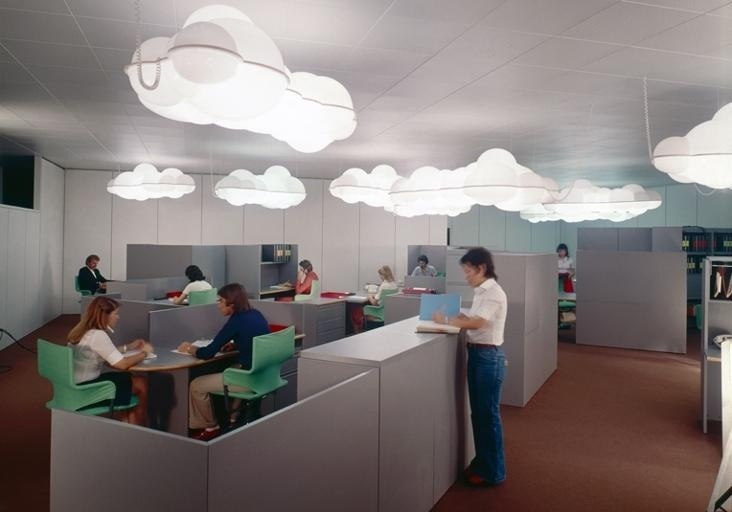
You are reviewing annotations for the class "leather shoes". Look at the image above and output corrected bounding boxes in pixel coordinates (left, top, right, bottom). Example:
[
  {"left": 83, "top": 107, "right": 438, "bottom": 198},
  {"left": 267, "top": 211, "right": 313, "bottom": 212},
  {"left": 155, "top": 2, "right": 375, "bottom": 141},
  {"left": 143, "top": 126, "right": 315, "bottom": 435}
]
[{"left": 193, "top": 428, "right": 220, "bottom": 440}]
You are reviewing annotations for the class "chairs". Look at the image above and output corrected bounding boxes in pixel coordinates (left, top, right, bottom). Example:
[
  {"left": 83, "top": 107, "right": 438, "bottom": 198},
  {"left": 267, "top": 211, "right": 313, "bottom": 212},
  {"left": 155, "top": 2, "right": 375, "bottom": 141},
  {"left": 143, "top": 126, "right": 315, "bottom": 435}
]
[
  {"left": 364, "top": 287, "right": 402, "bottom": 326},
  {"left": 294, "top": 279, "right": 321, "bottom": 303},
  {"left": 209, "top": 324, "right": 296, "bottom": 433},
  {"left": 188, "top": 286, "right": 219, "bottom": 308},
  {"left": 74, "top": 276, "right": 104, "bottom": 296},
  {"left": 36, "top": 337, "right": 139, "bottom": 422},
  {"left": 559, "top": 273, "right": 576, "bottom": 331}
]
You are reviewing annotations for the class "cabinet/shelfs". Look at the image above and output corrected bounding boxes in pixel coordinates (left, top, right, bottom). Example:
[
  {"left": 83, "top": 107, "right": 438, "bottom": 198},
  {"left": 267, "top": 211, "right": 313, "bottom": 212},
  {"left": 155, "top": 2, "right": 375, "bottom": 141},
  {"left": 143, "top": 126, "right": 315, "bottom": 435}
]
[
  {"left": 702, "top": 257, "right": 732, "bottom": 432},
  {"left": 225, "top": 245, "right": 298, "bottom": 294},
  {"left": 680, "top": 230, "right": 732, "bottom": 300}
]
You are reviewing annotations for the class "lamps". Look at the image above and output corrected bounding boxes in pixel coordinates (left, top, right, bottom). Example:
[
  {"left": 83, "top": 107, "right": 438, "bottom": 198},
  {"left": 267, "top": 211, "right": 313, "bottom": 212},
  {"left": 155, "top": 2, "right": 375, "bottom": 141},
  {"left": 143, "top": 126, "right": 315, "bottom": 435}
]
[
  {"left": 124, "top": 6, "right": 357, "bottom": 156},
  {"left": 328, "top": 149, "right": 661, "bottom": 224},
  {"left": 107, "top": 154, "right": 196, "bottom": 202},
  {"left": 642, "top": 83, "right": 732, "bottom": 191},
  {"left": 213, "top": 166, "right": 306, "bottom": 208}
]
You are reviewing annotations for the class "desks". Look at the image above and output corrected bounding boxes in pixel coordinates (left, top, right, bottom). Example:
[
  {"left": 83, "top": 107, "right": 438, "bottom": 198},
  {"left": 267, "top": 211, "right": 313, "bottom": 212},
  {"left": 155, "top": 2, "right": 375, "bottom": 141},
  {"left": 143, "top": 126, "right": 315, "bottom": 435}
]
[
  {"left": 100, "top": 329, "right": 306, "bottom": 435},
  {"left": 339, "top": 293, "right": 372, "bottom": 304},
  {"left": 148, "top": 294, "right": 222, "bottom": 308}
]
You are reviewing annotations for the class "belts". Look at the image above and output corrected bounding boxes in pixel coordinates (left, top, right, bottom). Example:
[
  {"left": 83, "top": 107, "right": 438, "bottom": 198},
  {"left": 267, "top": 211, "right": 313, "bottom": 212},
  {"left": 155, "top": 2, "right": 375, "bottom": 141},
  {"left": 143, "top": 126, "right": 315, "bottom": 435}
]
[{"left": 467, "top": 342, "right": 496, "bottom": 348}]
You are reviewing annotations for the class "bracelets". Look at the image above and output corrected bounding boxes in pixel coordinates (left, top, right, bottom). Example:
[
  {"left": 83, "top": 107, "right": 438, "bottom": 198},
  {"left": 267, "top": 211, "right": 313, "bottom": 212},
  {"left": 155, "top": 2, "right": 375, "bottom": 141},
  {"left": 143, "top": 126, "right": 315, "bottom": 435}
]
[
  {"left": 123, "top": 344, "right": 127, "bottom": 353},
  {"left": 444, "top": 316, "right": 448, "bottom": 324},
  {"left": 141, "top": 351, "right": 147, "bottom": 357},
  {"left": 186, "top": 344, "right": 192, "bottom": 351}
]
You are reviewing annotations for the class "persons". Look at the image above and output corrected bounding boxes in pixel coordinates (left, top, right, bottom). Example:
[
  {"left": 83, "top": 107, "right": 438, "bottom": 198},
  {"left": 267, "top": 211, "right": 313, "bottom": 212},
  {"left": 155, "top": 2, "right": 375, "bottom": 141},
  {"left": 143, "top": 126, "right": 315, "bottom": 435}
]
[
  {"left": 173, "top": 264, "right": 213, "bottom": 306},
  {"left": 78, "top": 254, "right": 107, "bottom": 295},
  {"left": 431, "top": 246, "right": 508, "bottom": 488},
  {"left": 555, "top": 243, "right": 576, "bottom": 293},
  {"left": 411, "top": 254, "right": 437, "bottom": 278},
  {"left": 66, "top": 295, "right": 154, "bottom": 426},
  {"left": 274, "top": 260, "right": 319, "bottom": 301},
  {"left": 351, "top": 265, "right": 398, "bottom": 333},
  {"left": 176, "top": 282, "right": 271, "bottom": 442}
]
